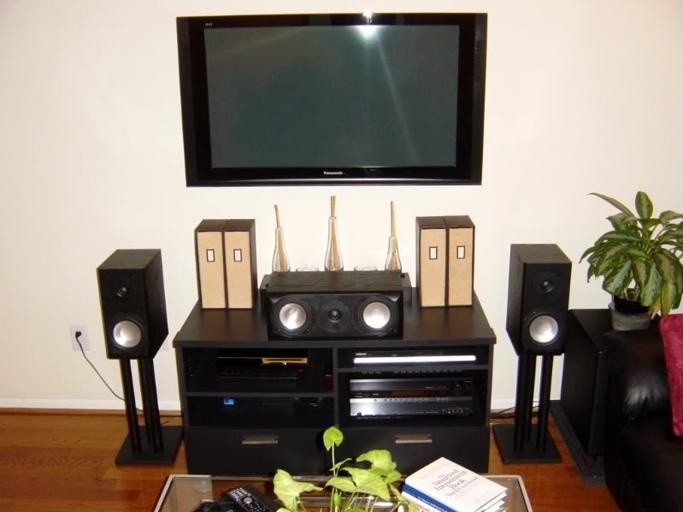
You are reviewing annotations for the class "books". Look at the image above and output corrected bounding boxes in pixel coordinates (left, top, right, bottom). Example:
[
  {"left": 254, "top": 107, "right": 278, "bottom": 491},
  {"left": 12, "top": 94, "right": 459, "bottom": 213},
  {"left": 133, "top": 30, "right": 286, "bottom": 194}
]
[{"left": 401, "top": 457, "right": 507, "bottom": 512}]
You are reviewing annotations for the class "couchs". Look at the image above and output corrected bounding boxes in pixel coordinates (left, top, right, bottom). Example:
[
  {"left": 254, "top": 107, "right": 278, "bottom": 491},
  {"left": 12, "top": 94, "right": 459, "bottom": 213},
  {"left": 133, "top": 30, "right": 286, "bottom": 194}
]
[{"left": 605, "top": 358, "right": 671, "bottom": 510}]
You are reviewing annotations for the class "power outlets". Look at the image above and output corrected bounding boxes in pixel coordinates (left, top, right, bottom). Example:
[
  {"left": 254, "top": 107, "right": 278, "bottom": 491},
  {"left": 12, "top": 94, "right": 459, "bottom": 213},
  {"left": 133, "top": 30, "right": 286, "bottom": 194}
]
[{"left": 69, "top": 323, "right": 90, "bottom": 353}]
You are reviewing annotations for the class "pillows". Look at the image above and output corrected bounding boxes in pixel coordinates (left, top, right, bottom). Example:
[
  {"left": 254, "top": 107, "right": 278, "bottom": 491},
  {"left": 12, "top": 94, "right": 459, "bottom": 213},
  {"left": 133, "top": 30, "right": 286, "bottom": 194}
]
[{"left": 658, "top": 312, "right": 682, "bottom": 438}]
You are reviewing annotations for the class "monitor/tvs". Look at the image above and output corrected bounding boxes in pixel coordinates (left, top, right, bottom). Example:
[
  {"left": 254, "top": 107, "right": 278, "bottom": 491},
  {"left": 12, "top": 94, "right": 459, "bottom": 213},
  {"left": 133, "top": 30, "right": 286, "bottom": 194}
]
[{"left": 176, "top": 12, "right": 488, "bottom": 187}]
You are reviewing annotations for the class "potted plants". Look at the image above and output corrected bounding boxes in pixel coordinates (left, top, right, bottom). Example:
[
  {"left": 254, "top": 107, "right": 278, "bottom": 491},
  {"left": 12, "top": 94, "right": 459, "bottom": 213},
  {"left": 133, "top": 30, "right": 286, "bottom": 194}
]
[{"left": 576, "top": 186, "right": 683, "bottom": 329}]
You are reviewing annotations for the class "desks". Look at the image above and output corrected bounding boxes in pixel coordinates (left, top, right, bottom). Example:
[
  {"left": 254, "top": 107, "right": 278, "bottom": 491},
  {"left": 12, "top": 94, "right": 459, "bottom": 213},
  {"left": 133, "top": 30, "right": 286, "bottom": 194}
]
[
  {"left": 561, "top": 306, "right": 666, "bottom": 458},
  {"left": 170, "top": 284, "right": 497, "bottom": 477}
]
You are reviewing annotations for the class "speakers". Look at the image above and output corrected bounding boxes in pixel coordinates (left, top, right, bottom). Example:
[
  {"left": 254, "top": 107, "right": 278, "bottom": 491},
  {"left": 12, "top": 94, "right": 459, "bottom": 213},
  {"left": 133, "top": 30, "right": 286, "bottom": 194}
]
[
  {"left": 96, "top": 248, "right": 169, "bottom": 359},
  {"left": 267, "top": 269, "right": 403, "bottom": 341},
  {"left": 506, "top": 243, "right": 571, "bottom": 355}
]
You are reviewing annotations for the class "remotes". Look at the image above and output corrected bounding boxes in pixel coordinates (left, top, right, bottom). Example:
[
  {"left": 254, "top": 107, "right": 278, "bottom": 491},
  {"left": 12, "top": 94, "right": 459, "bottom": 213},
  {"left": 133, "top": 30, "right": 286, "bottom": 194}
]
[{"left": 225, "top": 486, "right": 273, "bottom": 512}]
